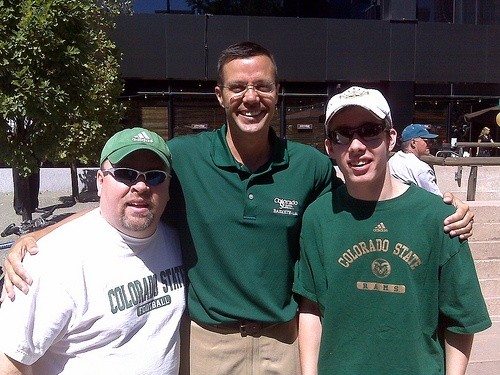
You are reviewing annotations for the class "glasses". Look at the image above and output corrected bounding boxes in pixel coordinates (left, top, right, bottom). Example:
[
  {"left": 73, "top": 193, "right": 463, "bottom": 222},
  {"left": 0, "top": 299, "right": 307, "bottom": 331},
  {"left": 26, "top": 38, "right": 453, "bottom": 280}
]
[
  {"left": 219, "top": 83, "right": 277, "bottom": 95},
  {"left": 416, "top": 138, "right": 429, "bottom": 144},
  {"left": 328, "top": 123, "right": 389, "bottom": 145},
  {"left": 102, "top": 168, "right": 171, "bottom": 186}
]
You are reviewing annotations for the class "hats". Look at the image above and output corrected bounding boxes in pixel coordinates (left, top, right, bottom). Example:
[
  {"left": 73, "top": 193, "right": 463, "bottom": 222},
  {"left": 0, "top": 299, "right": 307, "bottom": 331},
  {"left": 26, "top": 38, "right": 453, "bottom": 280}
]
[
  {"left": 325, "top": 86, "right": 393, "bottom": 135},
  {"left": 99, "top": 127, "right": 172, "bottom": 170},
  {"left": 401, "top": 124, "right": 438, "bottom": 141}
]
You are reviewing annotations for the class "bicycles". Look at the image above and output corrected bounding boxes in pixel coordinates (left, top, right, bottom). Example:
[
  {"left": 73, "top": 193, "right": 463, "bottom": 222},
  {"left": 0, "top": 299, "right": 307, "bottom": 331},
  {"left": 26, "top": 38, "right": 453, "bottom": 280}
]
[{"left": 0, "top": 205, "right": 67, "bottom": 306}]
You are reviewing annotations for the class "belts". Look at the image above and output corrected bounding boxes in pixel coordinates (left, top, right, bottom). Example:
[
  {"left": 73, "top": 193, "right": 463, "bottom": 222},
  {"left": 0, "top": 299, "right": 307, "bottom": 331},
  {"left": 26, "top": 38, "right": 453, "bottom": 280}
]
[{"left": 192, "top": 317, "right": 279, "bottom": 337}]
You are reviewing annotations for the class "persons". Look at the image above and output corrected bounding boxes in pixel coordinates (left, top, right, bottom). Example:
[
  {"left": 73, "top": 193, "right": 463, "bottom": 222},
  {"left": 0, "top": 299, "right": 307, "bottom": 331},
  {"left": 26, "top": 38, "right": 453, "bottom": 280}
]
[
  {"left": 1, "top": 126, "right": 184, "bottom": 375},
  {"left": 289, "top": 86, "right": 500, "bottom": 375},
  {"left": 0, "top": 42, "right": 476, "bottom": 375}
]
